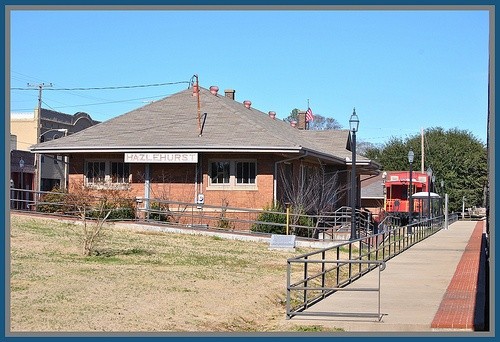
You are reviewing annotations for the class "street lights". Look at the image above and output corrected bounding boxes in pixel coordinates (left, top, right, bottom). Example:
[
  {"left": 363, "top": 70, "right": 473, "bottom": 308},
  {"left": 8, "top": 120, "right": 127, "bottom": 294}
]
[
  {"left": 439, "top": 179, "right": 444, "bottom": 222},
  {"left": 348, "top": 107, "right": 361, "bottom": 241},
  {"left": 432, "top": 174, "right": 435, "bottom": 223},
  {"left": 408, "top": 146, "right": 415, "bottom": 234},
  {"left": 426, "top": 165, "right": 433, "bottom": 228}
]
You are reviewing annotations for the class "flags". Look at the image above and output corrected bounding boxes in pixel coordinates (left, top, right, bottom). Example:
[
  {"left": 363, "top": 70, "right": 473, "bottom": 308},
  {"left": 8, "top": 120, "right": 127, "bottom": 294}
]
[{"left": 306, "top": 107, "right": 313, "bottom": 121}]
